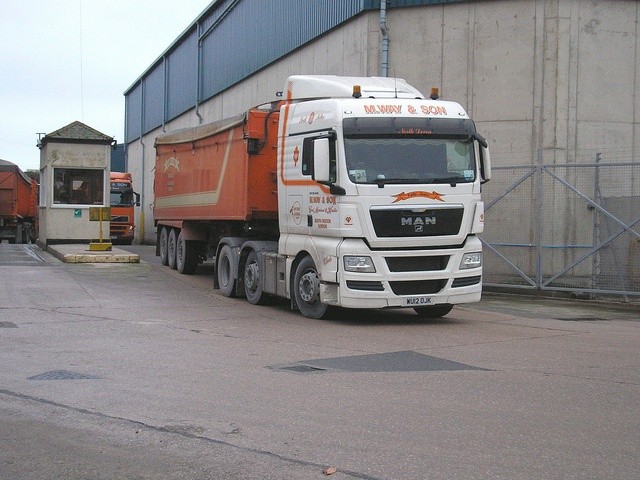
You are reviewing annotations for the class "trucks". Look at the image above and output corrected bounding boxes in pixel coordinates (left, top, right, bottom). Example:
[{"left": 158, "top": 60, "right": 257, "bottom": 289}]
[
  {"left": 1, "top": 160, "right": 40, "bottom": 244},
  {"left": 110, "top": 171, "right": 140, "bottom": 245},
  {"left": 150, "top": 76, "right": 492, "bottom": 318}
]
